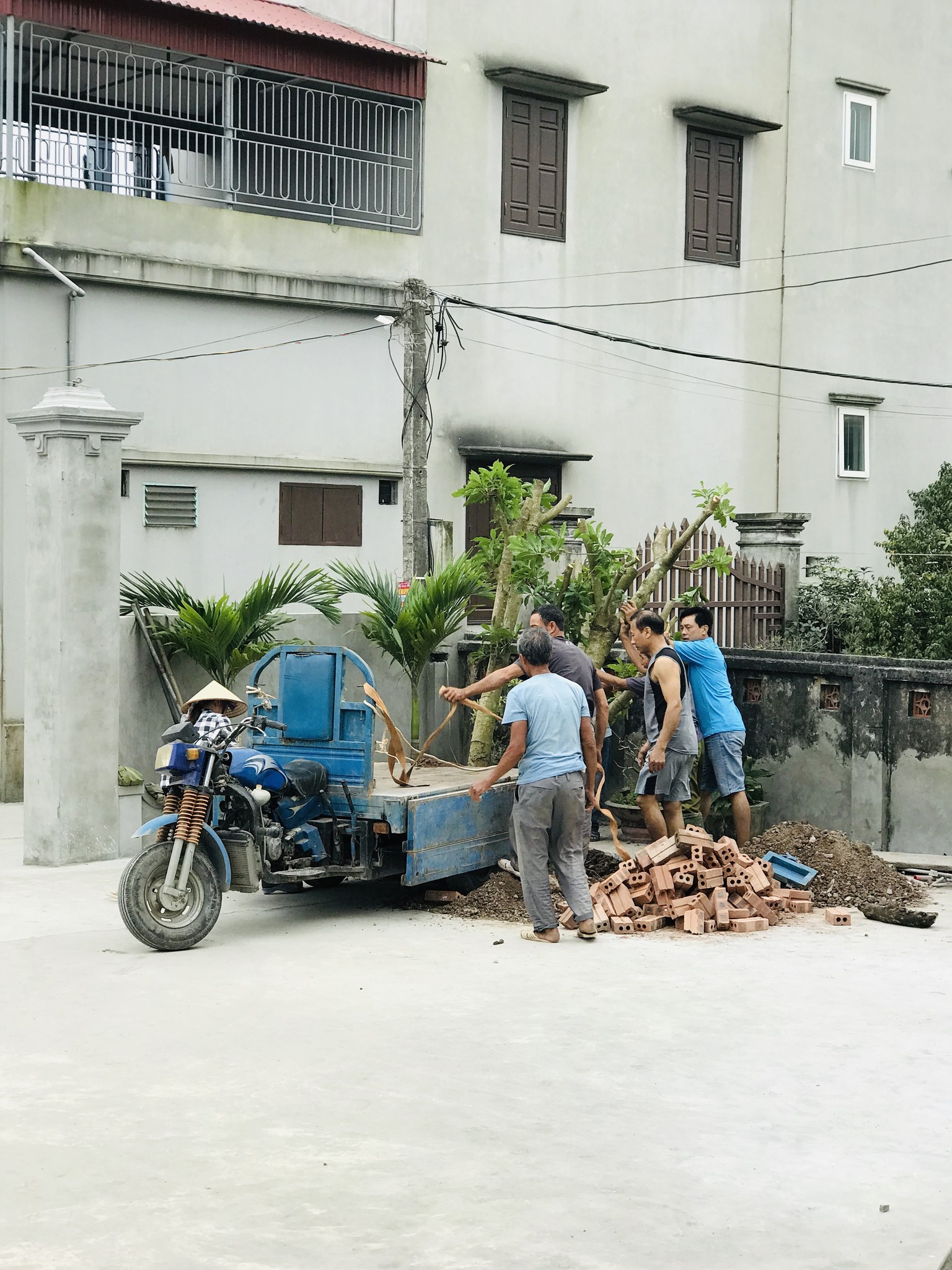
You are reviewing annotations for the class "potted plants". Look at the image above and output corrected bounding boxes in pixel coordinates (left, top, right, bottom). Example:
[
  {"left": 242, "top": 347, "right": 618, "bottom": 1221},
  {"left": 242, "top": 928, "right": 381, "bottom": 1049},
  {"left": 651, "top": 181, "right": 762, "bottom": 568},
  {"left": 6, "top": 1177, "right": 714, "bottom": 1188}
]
[
  {"left": 705, "top": 758, "right": 776, "bottom": 845},
  {"left": 605, "top": 725, "right": 664, "bottom": 843}
]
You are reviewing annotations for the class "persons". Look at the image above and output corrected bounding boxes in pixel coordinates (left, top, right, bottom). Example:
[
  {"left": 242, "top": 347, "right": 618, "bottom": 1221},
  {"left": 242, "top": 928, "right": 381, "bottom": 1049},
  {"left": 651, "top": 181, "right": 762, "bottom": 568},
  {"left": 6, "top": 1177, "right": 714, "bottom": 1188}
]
[
  {"left": 468, "top": 627, "right": 598, "bottom": 944},
  {"left": 439, "top": 605, "right": 610, "bottom": 876},
  {"left": 590, "top": 705, "right": 612, "bottom": 843},
  {"left": 594, "top": 611, "right": 698, "bottom": 842},
  {"left": 663, "top": 606, "right": 752, "bottom": 848},
  {"left": 594, "top": 618, "right": 703, "bottom": 743}
]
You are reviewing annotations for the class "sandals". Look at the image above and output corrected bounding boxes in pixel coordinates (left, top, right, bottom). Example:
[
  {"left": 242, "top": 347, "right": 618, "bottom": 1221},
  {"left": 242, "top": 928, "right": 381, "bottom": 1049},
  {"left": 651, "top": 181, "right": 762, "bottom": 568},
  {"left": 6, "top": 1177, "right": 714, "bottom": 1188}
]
[{"left": 590, "top": 831, "right": 601, "bottom": 842}]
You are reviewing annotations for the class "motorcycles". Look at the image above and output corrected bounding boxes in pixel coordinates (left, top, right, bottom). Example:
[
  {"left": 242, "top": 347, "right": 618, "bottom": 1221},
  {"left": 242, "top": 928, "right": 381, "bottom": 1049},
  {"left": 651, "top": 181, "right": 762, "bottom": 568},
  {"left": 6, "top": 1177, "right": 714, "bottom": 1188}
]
[{"left": 118, "top": 643, "right": 519, "bottom": 953}]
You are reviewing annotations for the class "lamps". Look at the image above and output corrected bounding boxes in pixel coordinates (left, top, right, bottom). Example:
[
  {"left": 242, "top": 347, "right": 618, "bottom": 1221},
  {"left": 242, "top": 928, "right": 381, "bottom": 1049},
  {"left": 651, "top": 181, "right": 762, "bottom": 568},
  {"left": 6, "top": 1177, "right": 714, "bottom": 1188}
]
[{"left": 374, "top": 314, "right": 404, "bottom": 328}]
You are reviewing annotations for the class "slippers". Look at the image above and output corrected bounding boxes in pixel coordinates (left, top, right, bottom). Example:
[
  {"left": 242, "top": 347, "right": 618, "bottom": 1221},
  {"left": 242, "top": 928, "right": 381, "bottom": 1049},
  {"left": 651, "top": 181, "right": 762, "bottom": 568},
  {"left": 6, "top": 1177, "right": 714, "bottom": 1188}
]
[
  {"left": 498, "top": 858, "right": 521, "bottom": 877},
  {"left": 520, "top": 927, "right": 551, "bottom": 943},
  {"left": 577, "top": 927, "right": 598, "bottom": 938}
]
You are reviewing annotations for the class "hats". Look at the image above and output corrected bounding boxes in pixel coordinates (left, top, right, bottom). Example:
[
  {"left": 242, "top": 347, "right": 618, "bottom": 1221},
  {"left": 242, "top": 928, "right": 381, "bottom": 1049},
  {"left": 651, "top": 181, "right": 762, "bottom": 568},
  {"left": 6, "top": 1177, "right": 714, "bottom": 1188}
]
[{"left": 181, "top": 680, "right": 248, "bottom": 718}]
